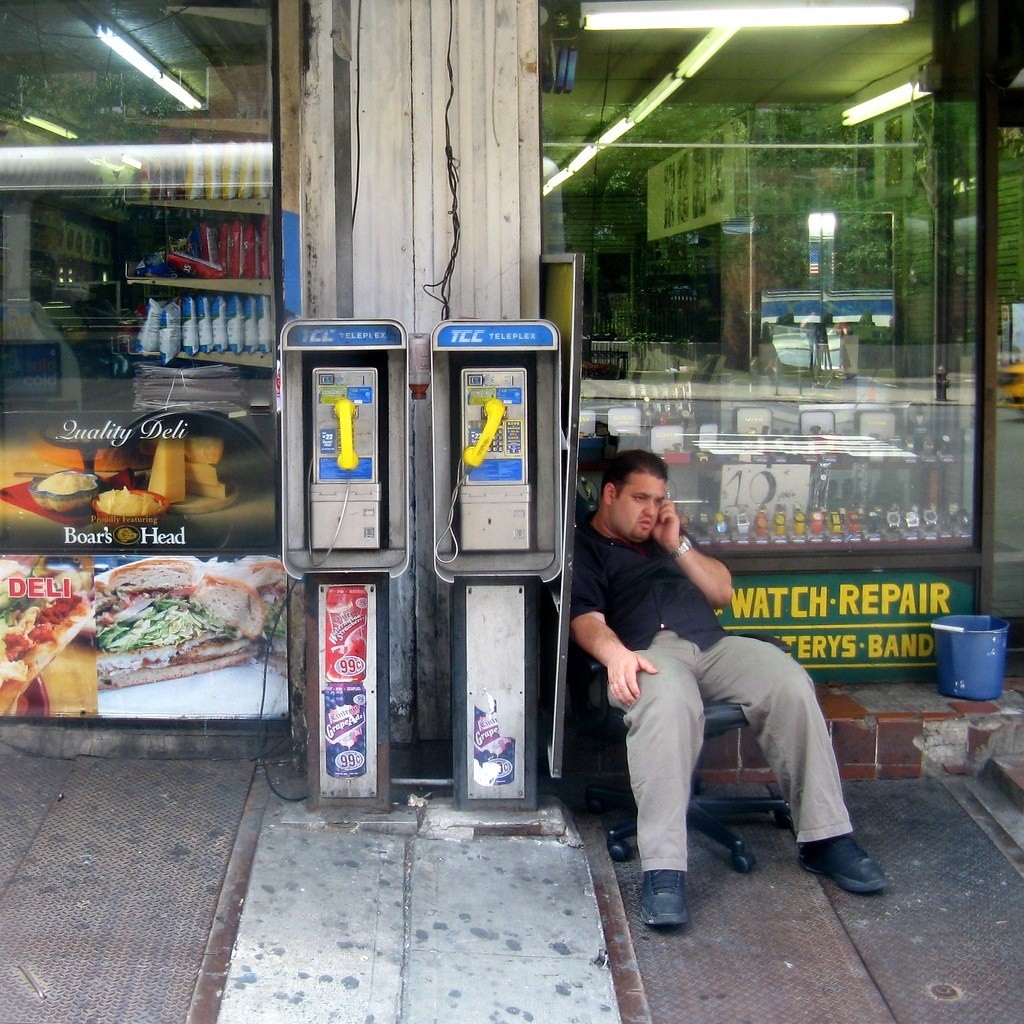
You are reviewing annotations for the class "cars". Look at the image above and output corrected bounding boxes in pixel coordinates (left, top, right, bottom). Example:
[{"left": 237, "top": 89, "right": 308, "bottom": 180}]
[{"left": 2, "top": 298, "right": 87, "bottom": 416}]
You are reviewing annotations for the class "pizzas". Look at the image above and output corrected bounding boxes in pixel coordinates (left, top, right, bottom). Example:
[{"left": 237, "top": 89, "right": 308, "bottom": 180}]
[{"left": 1, "top": 566, "right": 95, "bottom": 717}]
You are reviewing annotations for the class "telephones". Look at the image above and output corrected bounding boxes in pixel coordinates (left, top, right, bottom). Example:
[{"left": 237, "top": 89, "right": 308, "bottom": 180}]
[
  {"left": 458, "top": 367, "right": 532, "bottom": 551},
  {"left": 310, "top": 366, "right": 381, "bottom": 548}
]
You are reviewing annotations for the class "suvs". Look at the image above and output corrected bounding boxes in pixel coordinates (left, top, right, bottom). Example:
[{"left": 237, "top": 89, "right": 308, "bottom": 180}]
[{"left": 41, "top": 287, "right": 144, "bottom": 403}]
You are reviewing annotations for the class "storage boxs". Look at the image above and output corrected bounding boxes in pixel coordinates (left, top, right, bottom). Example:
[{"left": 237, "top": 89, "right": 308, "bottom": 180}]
[
  {"left": 648, "top": 423, "right": 690, "bottom": 464},
  {"left": 853, "top": 411, "right": 899, "bottom": 437},
  {"left": 696, "top": 422, "right": 720, "bottom": 453},
  {"left": 606, "top": 405, "right": 648, "bottom": 448},
  {"left": 579, "top": 410, "right": 605, "bottom": 448},
  {"left": 798, "top": 409, "right": 836, "bottom": 434}
]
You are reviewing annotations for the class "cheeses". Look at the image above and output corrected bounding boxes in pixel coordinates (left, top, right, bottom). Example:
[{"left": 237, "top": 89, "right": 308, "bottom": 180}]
[{"left": 145, "top": 430, "right": 237, "bottom": 504}]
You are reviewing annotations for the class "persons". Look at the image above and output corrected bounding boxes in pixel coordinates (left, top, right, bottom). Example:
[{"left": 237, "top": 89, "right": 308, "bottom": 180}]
[{"left": 568, "top": 448, "right": 886, "bottom": 926}]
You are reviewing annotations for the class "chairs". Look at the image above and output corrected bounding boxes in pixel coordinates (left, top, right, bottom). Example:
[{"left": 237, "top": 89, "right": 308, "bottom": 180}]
[{"left": 568, "top": 635, "right": 793, "bottom": 872}]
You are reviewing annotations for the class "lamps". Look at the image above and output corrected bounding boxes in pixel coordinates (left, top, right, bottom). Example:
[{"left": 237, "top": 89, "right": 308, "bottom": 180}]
[
  {"left": 592, "top": 111, "right": 635, "bottom": 149},
  {"left": 153, "top": 69, "right": 202, "bottom": 109},
  {"left": 578, "top": 0, "right": 915, "bottom": 31},
  {"left": 568, "top": 136, "right": 598, "bottom": 173},
  {"left": 675, "top": 27, "right": 744, "bottom": 78},
  {"left": 626, "top": 71, "right": 685, "bottom": 124},
  {"left": 94, "top": 22, "right": 163, "bottom": 79}
]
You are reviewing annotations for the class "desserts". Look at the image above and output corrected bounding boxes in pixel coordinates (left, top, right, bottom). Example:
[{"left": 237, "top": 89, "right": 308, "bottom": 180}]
[{"left": 28, "top": 471, "right": 170, "bottom": 533}]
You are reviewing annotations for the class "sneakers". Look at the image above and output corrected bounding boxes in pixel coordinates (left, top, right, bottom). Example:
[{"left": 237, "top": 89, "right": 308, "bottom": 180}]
[
  {"left": 640, "top": 870, "right": 689, "bottom": 924},
  {"left": 798, "top": 833, "right": 888, "bottom": 891}
]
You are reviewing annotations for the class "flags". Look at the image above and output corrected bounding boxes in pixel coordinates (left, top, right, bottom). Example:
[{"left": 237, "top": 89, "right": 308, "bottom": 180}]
[{"left": 810, "top": 250, "right": 834, "bottom": 274}]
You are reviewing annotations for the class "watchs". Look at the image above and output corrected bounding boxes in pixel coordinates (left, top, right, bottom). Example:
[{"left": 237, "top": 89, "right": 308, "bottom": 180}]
[
  {"left": 680, "top": 502, "right": 971, "bottom": 537},
  {"left": 743, "top": 426, "right": 902, "bottom": 453},
  {"left": 902, "top": 432, "right": 951, "bottom": 455},
  {"left": 670, "top": 536, "right": 692, "bottom": 559},
  {"left": 630, "top": 380, "right": 694, "bottom": 434}
]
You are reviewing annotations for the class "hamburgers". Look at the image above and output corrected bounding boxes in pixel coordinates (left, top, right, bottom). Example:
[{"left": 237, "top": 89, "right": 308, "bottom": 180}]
[{"left": 94, "top": 557, "right": 286, "bottom": 694}]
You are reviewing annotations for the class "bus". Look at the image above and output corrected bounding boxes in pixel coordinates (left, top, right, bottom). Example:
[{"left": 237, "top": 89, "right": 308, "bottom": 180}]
[{"left": 756, "top": 283, "right": 898, "bottom": 374}]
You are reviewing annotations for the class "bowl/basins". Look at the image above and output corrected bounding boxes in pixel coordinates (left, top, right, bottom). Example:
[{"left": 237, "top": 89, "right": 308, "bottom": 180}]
[
  {"left": 92, "top": 489, "right": 170, "bottom": 531},
  {"left": 29, "top": 470, "right": 100, "bottom": 514}
]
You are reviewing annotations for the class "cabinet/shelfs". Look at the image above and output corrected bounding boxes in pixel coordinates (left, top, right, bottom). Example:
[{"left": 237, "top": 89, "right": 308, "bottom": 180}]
[{"left": 124, "top": 0, "right": 275, "bottom": 371}]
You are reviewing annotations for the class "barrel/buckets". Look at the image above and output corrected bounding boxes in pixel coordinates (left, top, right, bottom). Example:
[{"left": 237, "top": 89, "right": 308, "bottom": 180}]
[{"left": 928, "top": 614, "right": 1010, "bottom": 701}]
